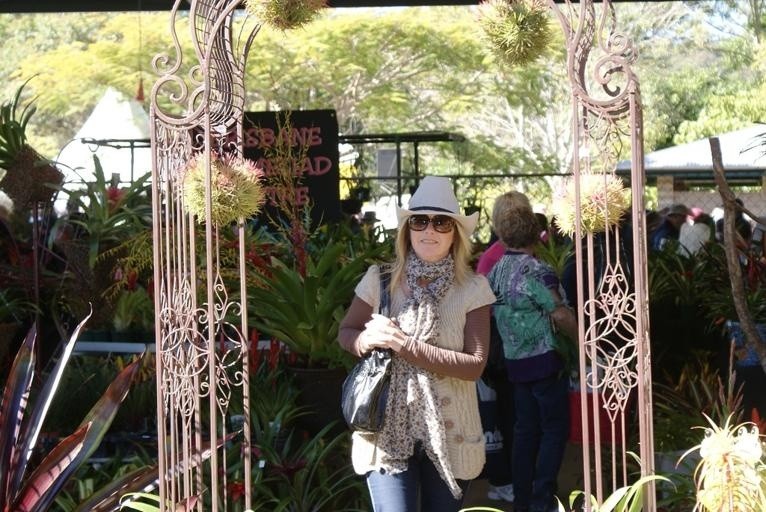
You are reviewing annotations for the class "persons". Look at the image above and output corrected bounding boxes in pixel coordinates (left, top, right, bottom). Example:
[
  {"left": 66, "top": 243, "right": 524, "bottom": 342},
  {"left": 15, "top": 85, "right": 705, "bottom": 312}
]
[
  {"left": 487, "top": 206, "right": 577, "bottom": 511},
  {"left": 533, "top": 198, "right": 766, "bottom": 313},
  {"left": 475, "top": 190, "right": 530, "bottom": 501},
  {"left": 339, "top": 175, "right": 496, "bottom": 512},
  {"left": 39, "top": 197, "right": 88, "bottom": 232},
  {"left": 360, "top": 211, "right": 381, "bottom": 228}
]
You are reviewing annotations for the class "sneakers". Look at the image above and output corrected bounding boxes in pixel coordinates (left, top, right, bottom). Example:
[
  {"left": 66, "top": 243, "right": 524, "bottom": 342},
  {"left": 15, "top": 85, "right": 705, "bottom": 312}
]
[{"left": 488, "top": 483, "right": 514, "bottom": 502}]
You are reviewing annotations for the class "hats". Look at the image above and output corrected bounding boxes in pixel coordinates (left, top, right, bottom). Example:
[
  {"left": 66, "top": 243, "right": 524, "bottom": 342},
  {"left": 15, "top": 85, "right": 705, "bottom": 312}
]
[
  {"left": 396, "top": 176, "right": 480, "bottom": 233},
  {"left": 666, "top": 203, "right": 688, "bottom": 217}
]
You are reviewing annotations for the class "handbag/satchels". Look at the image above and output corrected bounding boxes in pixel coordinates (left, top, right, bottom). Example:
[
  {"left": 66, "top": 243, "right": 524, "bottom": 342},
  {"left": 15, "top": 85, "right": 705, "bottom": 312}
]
[{"left": 340, "top": 264, "right": 395, "bottom": 433}]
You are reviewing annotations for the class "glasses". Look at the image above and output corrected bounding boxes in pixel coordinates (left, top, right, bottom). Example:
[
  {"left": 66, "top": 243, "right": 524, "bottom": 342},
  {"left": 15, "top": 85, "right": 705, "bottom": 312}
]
[{"left": 406, "top": 214, "right": 456, "bottom": 234}]
